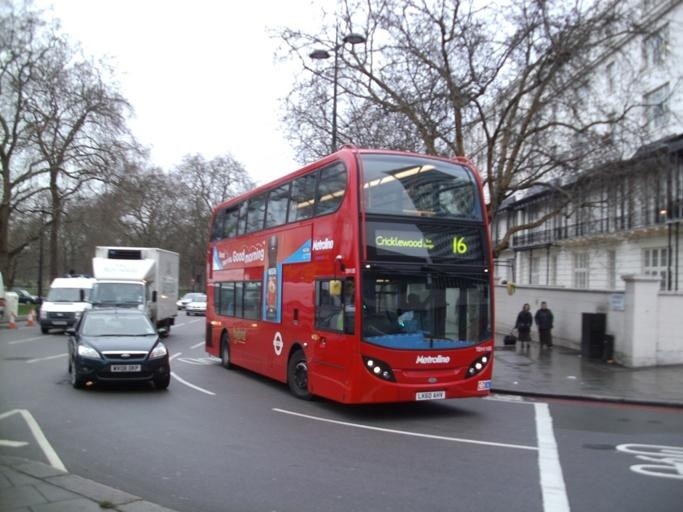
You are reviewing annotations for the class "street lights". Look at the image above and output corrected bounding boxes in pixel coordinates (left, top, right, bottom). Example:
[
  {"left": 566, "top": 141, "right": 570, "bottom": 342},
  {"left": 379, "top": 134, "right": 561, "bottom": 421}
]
[{"left": 308, "top": 31, "right": 369, "bottom": 154}]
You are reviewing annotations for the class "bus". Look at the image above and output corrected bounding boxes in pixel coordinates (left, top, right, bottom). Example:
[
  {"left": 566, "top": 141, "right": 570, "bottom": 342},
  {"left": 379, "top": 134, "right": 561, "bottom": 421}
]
[{"left": 203, "top": 143, "right": 515, "bottom": 406}]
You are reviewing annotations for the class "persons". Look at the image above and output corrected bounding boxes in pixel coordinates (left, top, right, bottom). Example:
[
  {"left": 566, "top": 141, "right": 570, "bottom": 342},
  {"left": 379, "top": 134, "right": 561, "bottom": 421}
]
[
  {"left": 534, "top": 300, "right": 556, "bottom": 350},
  {"left": 511, "top": 302, "right": 534, "bottom": 349},
  {"left": 398, "top": 293, "right": 428, "bottom": 315}
]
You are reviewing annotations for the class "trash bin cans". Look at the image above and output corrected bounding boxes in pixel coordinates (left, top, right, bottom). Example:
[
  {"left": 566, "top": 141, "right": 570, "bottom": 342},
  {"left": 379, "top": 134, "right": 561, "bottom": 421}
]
[{"left": 581, "top": 313, "right": 606, "bottom": 358}]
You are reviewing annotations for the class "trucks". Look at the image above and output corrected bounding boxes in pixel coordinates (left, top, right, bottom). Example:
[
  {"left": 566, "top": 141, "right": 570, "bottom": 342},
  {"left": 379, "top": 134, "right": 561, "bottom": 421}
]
[{"left": 90, "top": 245, "right": 180, "bottom": 338}]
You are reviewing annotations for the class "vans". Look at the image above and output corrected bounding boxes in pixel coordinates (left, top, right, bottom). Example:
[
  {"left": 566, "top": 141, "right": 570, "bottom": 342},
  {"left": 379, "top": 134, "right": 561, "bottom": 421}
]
[
  {"left": 38, "top": 273, "right": 97, "bottom": 337},
  {"left": 0, "top": 271, "right": 6, "bottom": 322}
]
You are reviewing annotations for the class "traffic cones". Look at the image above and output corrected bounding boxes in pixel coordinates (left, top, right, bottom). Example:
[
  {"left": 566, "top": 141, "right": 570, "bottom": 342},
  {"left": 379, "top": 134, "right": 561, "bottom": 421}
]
[
  {"left": 4, "top": 310, "right": 17, "bottom": 330},
  {"left": 23, "top": 309, "right": 34, "bottom": 327}
]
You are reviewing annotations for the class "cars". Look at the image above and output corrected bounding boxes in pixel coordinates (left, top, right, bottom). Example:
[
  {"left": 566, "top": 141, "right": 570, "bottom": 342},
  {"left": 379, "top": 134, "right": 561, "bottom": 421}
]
[
  {"left": 185, "top": 294, "right": 206, "bottom": 317},
  {"left": 11, "top": 287, "right": 38, "bottom": 305},
  {"left": 62, "top": 301, "right": 173, "bottom": 392},
  {"left": 174, "top": 291, "right": 204, "bottom": 310}
]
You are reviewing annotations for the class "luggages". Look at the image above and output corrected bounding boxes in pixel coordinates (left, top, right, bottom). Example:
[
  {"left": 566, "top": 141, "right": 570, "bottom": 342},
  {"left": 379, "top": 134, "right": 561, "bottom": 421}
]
[{"left": 503, "top": 327, "right": 516, "bottom": 345}]
